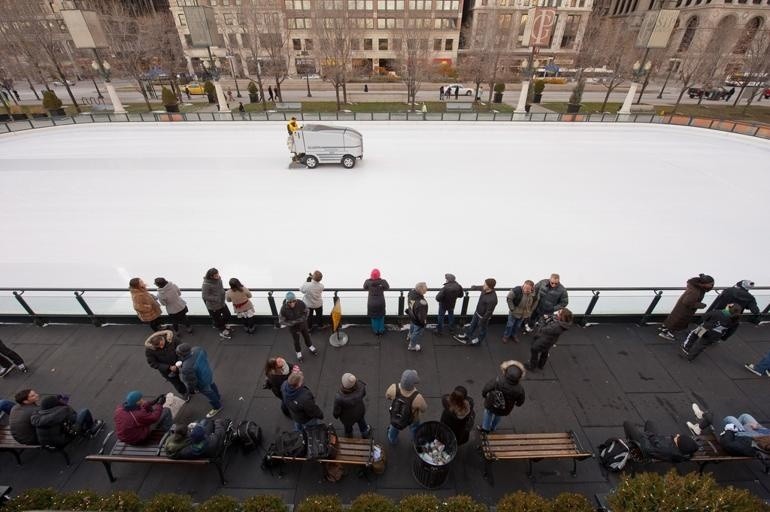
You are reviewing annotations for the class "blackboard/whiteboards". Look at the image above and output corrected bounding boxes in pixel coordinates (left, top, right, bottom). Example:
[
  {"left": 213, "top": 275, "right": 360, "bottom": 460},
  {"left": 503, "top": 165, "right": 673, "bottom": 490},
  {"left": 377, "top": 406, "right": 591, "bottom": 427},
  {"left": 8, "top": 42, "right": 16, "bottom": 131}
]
[{"left": 286, "top": 124, "right": 363, "bottom": 168}]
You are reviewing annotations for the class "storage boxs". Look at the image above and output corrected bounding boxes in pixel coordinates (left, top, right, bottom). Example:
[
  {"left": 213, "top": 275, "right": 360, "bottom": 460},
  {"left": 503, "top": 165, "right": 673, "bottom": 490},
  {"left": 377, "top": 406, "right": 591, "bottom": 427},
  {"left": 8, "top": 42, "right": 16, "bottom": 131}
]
[
  {"left": 0, "top": 414, "right": 71, "bottom": 467},
  {"left": 83, "top": 421, "right": 237, "bottom": 485},
  {"left": 265, "top": 435, "right": 376, "bottom": 483},
  {"left": 597, "top": 421, "right": 770, "bottom": 480},
  {"left": 474, "top": 424, "right": 596, "bottom": 488}
]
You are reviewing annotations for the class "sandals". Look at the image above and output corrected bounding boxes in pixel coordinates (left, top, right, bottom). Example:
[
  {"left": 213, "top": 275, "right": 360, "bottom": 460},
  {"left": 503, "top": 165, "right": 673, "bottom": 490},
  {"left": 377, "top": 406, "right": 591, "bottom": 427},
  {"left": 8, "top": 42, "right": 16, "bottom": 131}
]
[
  {"left": 725, "top": 70, "right": 768, "bottom": 88},
  {"left": 532, "top": 67, "right": 613, "bottom": 85}
]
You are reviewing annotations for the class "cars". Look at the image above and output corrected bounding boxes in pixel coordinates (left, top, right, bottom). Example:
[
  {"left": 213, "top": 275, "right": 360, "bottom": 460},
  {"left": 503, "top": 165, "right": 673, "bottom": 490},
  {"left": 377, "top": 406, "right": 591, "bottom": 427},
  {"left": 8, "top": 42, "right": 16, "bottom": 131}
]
[{"left": 175, "top": 359, "right": 183, "bottom": 369}]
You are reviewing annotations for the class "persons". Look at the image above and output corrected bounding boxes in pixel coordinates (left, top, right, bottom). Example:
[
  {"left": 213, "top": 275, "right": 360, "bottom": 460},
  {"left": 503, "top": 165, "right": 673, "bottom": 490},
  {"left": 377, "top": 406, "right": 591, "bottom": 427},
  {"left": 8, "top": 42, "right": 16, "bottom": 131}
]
[
  {"left": 686, "top": 403, "right": 769, "bottom": 461},
  {"left": 287, "top": 117, "right": 304, "bottom": 135},
  {"left": 764, "top": 86, "right": 770, "bottom": 99},
  {"left": 455, "top": 86, "right": 459, "bottom": 100},
  {"left": 260, "top": 89, "right": 265, "bottom": 103},
  {"left": 155, "top": 277, "right": 192, "bottom": 337},
  {"left": 226, "top": 278, "right": 256, "bottom": 332},
  {"left": 185, "top": 88, "right": 191, "bottom": 100},
  {"left": 447, "top": 87, "right": 452, "bottom": 99},
  {"left": 452, "top": 278, "right": 498, "bottom": 345},
  {"left": 744, "top": 357, "right": 769, "bottom": 376},
  {"left": 145, "top": 331, "right": 191, "bottom": 401},
  {"left": 405, "top": 282, "right": 428, "bottom": 351},
  {"left": 239, "top": 102, "right": 247, "bottom": 120},
  {"left": 622, "top": 418, "right": 699, "bottom": 460},
  {"left": 440, "top": 86, "right": 444, "bottom": 100},
  {"left": 658, "top": 274, "right": 714, "bottom": 340},
  {"left": 177, "top": 343, "right": 223, "bottom": 418},
  {"left": 202, "top": 268, "right": 233, "bottom": 339},
  {"left": 384, "top": 369, "right": 428, "bottom": 444},
  {"left": 283, "top": 372, "right": 324, "bottom": 432},
  {"left": 680, "top": 303, "right": 741, "bottom": 362},
  {"left": 440, "top": 386, "right": 475, "bottom": 446},
  {"left": 30, "top": 395, "right": 103, "bottom": 447},
  {"left": 280, "top": 293, "right": 317, "bottom": 360},
  {"left": 274, "top": 85, "right": 280, "bottom": 100},
  {"left": 503, "top": 280, "right": 540, "bottom": 343},
  {"left": 332, "top": 372, "right": 371, "bottom": 440},
  {"left": 714, "top": 280, "right": 759, "bottom": 314},
  {"left": 476, "top": 86, "right": 482, "bottom": 100},
  {"left": 1, "top": 340, "right": 26, "bottom": 375},
  {"left": 725, "top": 87, "right": 735, "bottom": 101},
  {"left": 268, "top": 85, "right": 273, "bottom": 101},
  {"left": 262, "top": 355, "right": 301, "bottom": 420},
  {"left": 301, "top": 270, "right": 325, "bottom": 332},
  {"left": 523, "top": 307, "right": 573, "bottom": 371},
  {"left": 164, "top": 416, "right": 232, "bottom": 460},
  {"left": 434, "top": 274, "right": 464, "bottom": 335},
  {"left": 13, "top": 91, "right": 21, "bottom": 101},
  {"left": 9, "top": 388, "right": 44, "bottom": 446},
  {"left": 525, "top": 274, "right": 568, "bottom": 331},
  {"left": 364, "top": 269, "right": 389, "bottom": 335},
  {"left": 130, "top": 278, "right": 162, "bottom": 332},
  {"left": 226, "top": 88, "right": 235, "bottom": 102},
  {"left": 364, "top": 85, "right": 368, "bottom": 92},
  {"left": 114, "top": 392, "right": 172, "bottom": 446},
  {"left": 477, "top": 360, "right": 526, "bottom": 433},
  {"left": 422, "top": 101, "right": 428, "bottom": 120}
]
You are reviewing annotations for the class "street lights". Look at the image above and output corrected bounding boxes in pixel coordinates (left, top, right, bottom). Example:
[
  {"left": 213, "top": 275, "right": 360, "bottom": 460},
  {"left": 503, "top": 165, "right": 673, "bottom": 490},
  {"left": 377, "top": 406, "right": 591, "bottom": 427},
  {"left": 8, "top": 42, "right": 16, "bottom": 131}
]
[
  {"left": 236, "top": 420, "right": 262, "bottom": 452},
  {"left": 485, "top": 389, "right": 507, "bottom": 412},
  {"left": 303, "top": 419, "right": 340, "bottom": 461},
  {"left": 373, "top": 441, "right": 388, "bottom": 477},
  {"left": 264, "top": 422, "right": 305, "bottom": 471}
]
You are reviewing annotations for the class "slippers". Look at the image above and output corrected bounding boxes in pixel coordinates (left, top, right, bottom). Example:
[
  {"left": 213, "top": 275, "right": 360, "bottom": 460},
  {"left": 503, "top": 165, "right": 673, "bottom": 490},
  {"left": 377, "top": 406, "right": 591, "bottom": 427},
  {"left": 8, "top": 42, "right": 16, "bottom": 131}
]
[
  {"left": 598, "top": 436, "right": 630, "bottom": 472},
  {"left": 390, "top": 384, "right": 419, "bottom": 430},
  {"left": 705, "top": 323, "right": 731, "bottom": 343}
]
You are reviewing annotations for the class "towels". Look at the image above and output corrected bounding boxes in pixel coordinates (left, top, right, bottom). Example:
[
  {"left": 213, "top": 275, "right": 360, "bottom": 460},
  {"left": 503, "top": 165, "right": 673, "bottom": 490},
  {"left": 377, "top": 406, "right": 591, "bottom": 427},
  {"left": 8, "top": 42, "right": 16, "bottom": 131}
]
[{"left": 412, "top": 420, "right": 458, "bottom": 491}]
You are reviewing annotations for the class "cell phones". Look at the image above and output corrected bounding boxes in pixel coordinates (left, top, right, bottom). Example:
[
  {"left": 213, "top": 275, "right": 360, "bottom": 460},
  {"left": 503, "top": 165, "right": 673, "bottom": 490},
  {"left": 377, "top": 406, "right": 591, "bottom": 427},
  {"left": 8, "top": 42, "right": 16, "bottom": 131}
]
[{"left": 307, "top": 272, "right": 313, "bottom": 282}]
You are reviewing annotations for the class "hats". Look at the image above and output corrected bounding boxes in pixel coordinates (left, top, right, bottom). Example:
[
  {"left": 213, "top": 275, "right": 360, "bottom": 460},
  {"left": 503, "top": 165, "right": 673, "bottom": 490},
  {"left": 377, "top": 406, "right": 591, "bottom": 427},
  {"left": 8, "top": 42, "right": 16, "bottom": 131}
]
[
  {"left": 181, "top": 80, "right": 209, "bottom": 96},
  {"left": 688, "top": 82, "right": 728, "bottom": 99},
  {"left": 54, "top": 79, "right": 76, "bottom": 87},
  {"left": 443, "top": 82, "right": 474, "bottom": 96}
]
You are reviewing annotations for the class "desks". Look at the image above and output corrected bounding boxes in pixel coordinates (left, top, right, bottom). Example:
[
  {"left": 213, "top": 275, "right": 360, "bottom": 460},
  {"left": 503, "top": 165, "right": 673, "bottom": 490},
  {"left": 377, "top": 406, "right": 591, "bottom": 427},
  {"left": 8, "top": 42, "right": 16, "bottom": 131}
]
[
  {"left": 202, "top": 59, "right": 233, "bottom": 121},
  {"left": 616, "top": 59, "right": 652, "bottom": 121},
  {"left": 656, "top": 56, "right": 681, "bottom": 100},
  {"left": 511, "top": 57, "right": 540, "bottom": 120},
  {"left": 91, "top": 59, "right": 130, "bottom": 122}
]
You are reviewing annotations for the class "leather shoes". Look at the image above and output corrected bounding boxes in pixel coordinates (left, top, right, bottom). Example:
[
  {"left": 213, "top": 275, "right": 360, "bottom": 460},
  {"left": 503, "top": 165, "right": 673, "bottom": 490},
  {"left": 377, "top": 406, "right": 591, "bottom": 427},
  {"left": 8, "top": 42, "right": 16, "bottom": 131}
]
[{"left": 725, "top": 423, "right": 739, "bottom": 433}]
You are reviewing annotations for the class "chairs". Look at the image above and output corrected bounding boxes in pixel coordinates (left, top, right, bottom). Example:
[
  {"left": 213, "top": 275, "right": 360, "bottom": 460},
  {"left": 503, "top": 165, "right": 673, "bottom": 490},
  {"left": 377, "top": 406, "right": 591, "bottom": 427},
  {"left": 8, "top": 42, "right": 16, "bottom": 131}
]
[
  {"left": 407, "top": 343, "right": 421, "bottom": 350},
  {"left": 90, "top": 419, "right": 103, "bottom": 438},
  {"left": 297, "top": 352, "right": 302, "bottom": 361},
  {"left": 745, "top": 362, "right": 761, "bottom": 376},
  {"left": 218, "top": 324, "right": 235, "bottom": 338},
  {"left": 502, "top": 332, "right": 521, "bottom": 343},
  {"left": 686, "top": 420, "right": 702, "bottom": 436},
  {"left": 0, "top": 364, "right": 29, "bottom": 378},
  {"left": 206, "top": 406, "right": 223, "bottom": 419},
  {"left": 309, "top": 345, "right": 317, "bottom": 356},
  {"left": 432, "top": 328, "right": 454, "bottom": 336},
  {"left": 659, "top": 329, "right": 676, "bottom": 341},
  {"left": 453, "top": 334, "right": 482, "bottom": 344},
  {"left": 692, "top": 402, "right": 703, "bottom": 420},
  {"left": 364, "top": 425, "right": 372, "bottom": 439}
]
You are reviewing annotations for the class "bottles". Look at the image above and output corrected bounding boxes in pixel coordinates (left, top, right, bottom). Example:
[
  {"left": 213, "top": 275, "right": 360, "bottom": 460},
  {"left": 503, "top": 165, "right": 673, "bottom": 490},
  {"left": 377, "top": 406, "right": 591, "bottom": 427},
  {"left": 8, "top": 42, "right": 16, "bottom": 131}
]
[
  {"left": 445, "top": 273, "right": 455, "bottom": 280},
  {"left": 287, "top": 292, "right": 294, "bottom": 299},
  {"left": 678, "top": 435, "right": 698, "bottom": 453},
  {"left": 127, "top": 392, "right": 142, "bottom": 405},
  {"left": 486, "top": 278, "right": 496, "bottom": 289},
  {"left": 699, "top": 274, "right": 713, "bottom": 284},
  {"left": 342, "top": 371, "right": 356, "bottom": 388},
  {"left": 739, "top": 278, "right": 755, "bottom": 290},
  {"left": 371, "top": 271, "right": 381, "bottom": 280}
]
